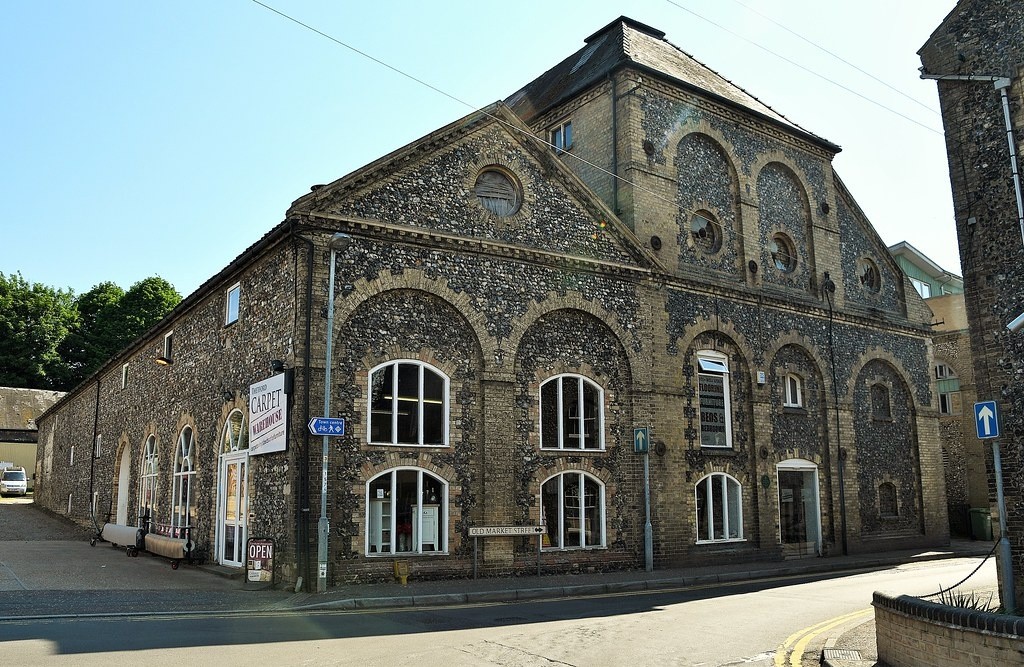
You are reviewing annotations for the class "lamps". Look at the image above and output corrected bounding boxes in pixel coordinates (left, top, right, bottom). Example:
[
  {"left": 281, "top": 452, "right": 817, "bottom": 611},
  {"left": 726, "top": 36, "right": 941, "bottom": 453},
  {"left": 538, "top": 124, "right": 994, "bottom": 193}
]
[{"left": 155, "top": 357, "right": 173, "bottom": 366}]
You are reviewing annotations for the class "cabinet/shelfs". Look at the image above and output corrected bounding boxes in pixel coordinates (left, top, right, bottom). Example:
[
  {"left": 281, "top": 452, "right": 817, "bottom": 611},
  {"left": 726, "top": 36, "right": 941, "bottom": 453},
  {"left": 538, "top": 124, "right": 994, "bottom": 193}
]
[
  {"left": 411, "top": 504, "right": 440, "bottom": 551},
  {"left": 370, "top": 498, "right": 391, "bottom": 552}
]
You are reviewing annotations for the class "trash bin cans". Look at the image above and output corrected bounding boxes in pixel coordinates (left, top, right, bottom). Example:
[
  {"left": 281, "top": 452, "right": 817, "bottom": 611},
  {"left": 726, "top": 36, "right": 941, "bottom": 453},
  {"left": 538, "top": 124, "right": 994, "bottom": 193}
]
[{"left": 970, "top": 507, "right": 992, "bottom": 541}]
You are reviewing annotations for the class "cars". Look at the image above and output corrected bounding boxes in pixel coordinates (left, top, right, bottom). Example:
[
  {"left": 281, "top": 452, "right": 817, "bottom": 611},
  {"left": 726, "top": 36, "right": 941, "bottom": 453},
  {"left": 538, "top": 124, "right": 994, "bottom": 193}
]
[{"left": 0, "top": 467, "right": 30, "bottom": 497}]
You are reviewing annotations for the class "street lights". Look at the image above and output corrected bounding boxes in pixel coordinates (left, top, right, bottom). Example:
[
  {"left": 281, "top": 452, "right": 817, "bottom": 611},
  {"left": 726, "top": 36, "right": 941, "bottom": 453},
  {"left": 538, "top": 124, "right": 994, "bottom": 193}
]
[{"left": 317, "top": 232, "right": 353, "bottom": 591}]
